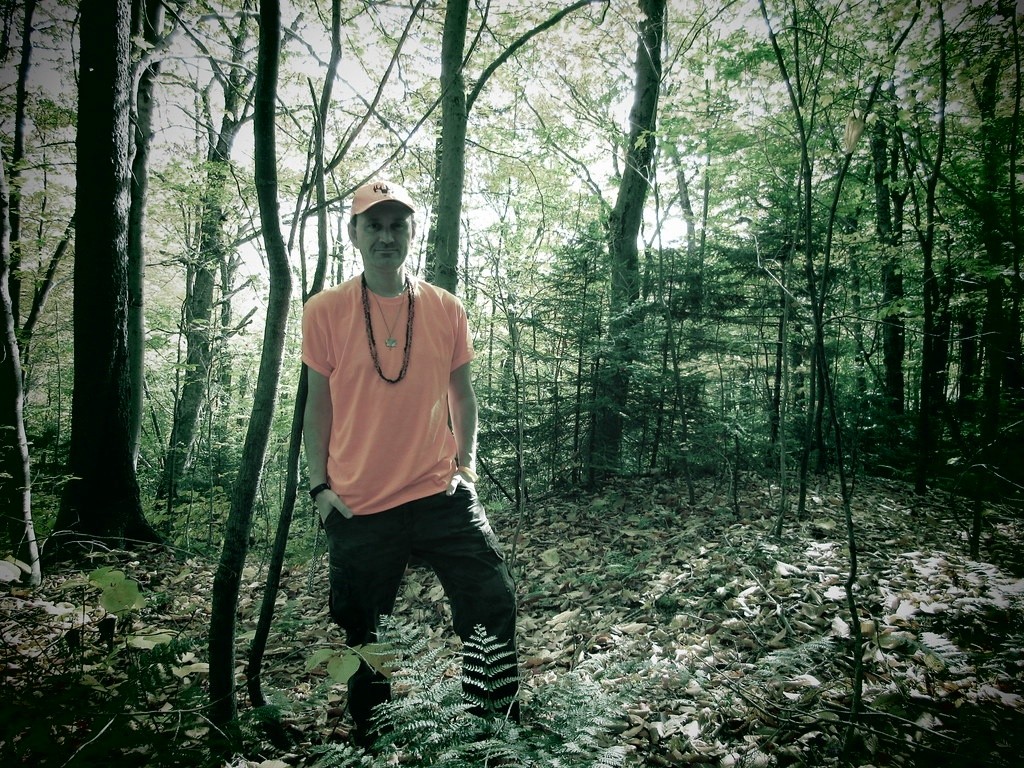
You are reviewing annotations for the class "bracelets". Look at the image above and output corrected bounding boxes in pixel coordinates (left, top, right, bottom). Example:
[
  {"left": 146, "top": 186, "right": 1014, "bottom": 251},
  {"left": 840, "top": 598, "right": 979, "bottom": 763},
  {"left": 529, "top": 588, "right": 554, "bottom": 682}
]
[
  {"left": 309, "top": 483, "right": 330, "bottom": 499},
  {"left": 459, "top": 466, "right": 478, "bottom": 482}
]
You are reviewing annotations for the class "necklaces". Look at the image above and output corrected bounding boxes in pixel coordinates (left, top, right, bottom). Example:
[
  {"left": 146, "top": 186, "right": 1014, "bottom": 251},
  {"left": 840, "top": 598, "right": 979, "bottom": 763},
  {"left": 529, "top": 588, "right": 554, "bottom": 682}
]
[{"left": 361, "top": 272, "right": 415, "bottom": 382}]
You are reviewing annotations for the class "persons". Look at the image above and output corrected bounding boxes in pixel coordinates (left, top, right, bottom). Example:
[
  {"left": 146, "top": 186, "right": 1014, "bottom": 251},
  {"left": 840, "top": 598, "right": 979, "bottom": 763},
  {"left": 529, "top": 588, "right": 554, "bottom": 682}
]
[{"left": 301, "top": 181, "right": 520, "bottom": 742}]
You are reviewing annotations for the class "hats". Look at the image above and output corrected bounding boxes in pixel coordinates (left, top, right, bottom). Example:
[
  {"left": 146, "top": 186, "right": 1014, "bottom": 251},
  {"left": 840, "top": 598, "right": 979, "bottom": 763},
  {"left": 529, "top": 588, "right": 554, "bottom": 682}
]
[{"left": 349, "top": 181, "right": 416, "bottom": 221}]
